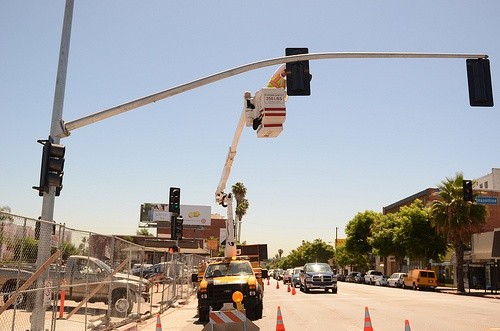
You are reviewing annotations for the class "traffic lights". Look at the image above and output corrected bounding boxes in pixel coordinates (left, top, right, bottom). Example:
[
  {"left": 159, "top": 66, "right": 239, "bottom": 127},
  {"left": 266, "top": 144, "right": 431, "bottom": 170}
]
[
  {"left": 462, "top": 180, "right": 473, "bottom": 201},
  {"left": 40, "top": 141, "right": 66, "bottom": 188},
  {"left": 173, "top": 216, "right": 183, "bottom": 239},
  {"left": 168, "top": 187, "right": 181, "bottom": 212},
  {"left": 169, "top": 246, "right": 180, "bottom": 255}
]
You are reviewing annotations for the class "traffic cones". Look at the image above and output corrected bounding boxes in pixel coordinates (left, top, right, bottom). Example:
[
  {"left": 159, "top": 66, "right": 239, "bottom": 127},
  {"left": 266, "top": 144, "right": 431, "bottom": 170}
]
[
  {"left": 267, "top": 278, "right": 270, "bottom": 285},
  {"left": 363, "top": 306, "right": 373, "bottom": 331},
  {"left": 276, "top": 306, "right": 286, "bottom": 331},
  {"left": 405, "top": 320, "right": 411, "bottom": 331},
  {"left": 276, "top": 280, "right": 280, "bottom": 289},
  {"left": 291, "top": 283, "right": 296, "bottom": 295},
  {"left": 155, "top": 313, "right": 163, "bottom": 331},
  {"left": 287, "top": 281, "right": 291, "bottom": 292}
]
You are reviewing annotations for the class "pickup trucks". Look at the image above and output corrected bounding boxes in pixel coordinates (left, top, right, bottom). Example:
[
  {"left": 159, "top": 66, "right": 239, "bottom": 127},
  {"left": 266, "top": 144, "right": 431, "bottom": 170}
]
[{"left": 2, "top": 253, "right": 149, "bottom": 317}]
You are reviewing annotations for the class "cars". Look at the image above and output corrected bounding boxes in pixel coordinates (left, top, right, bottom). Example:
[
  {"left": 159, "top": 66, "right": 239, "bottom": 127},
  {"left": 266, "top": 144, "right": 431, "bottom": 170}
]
[
  {"left": 353, "top": 273, "right": 365, "bottom": 284},
  {"left": 363, "top": 269, "right": 384, "bottom": 285},
  {"left": 298, "top": 262, "right": 337, "bottom": 294},
  {"left": 132, "top": 267, "right": 175, "bottom": 285},
  {"left": 0, "top": 266, "right": 36, "bottom": 309},
  {"left": 267, "top": 266, "right": 346, "bottom": 288},
  {"left": 386, "top": 272, "right": 406, "bottom": 288},
  {"left": 375, "top": 275, "right": 390, "bottom": 286},
  {"left": 345, "top": 271, "right": 360, "bottom": 282}
]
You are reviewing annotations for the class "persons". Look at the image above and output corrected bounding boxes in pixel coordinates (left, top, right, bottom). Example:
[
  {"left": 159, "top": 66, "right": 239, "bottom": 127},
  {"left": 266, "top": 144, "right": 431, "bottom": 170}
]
[{"left": 267, "top": 63, "right": 287, "bottom": 90}]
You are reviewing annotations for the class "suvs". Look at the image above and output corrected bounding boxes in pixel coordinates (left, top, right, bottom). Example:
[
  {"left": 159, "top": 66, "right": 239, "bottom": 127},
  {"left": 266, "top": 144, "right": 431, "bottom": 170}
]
[
  {"left": 195, "top": 256, "right": 264, "bottom": 324},
  {"left": 402, "top": 268, "right": 437, "bottom": 291}
]
